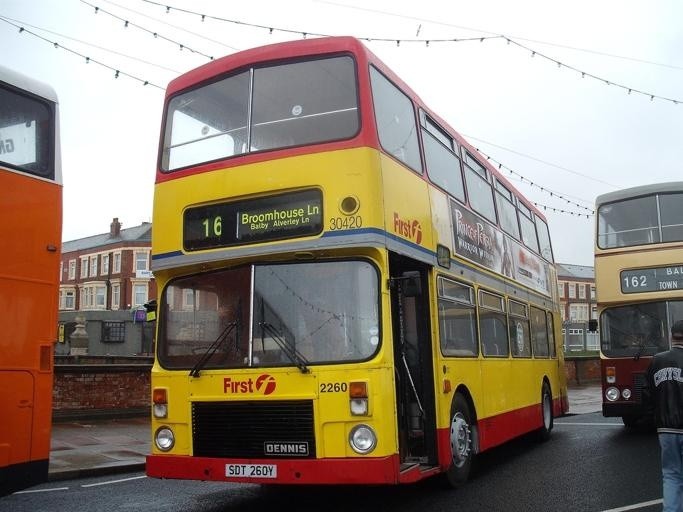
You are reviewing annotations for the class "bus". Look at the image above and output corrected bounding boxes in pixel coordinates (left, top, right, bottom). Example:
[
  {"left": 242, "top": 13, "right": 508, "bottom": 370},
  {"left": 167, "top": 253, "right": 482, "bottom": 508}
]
[
  {"left": 589, "top": 181, "right": 683, "bottom": 428},
  {"left": 0, "top": 66, "right": 62, "bottom": 495},
  {"left": 145, "top": 36, "right": 567, "bottom": 486}
]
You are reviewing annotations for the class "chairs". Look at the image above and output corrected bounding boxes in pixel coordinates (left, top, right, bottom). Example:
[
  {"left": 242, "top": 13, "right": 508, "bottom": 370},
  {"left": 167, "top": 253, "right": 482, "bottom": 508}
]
[{"left": 446, "top": 339, "right": 554, "bottom": 356}]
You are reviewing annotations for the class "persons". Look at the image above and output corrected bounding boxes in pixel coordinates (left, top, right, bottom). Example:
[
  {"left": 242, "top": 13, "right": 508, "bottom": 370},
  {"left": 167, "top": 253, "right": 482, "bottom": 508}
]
[{"left": 643, "top": 320, "right": 683, "bottom": 512}]
